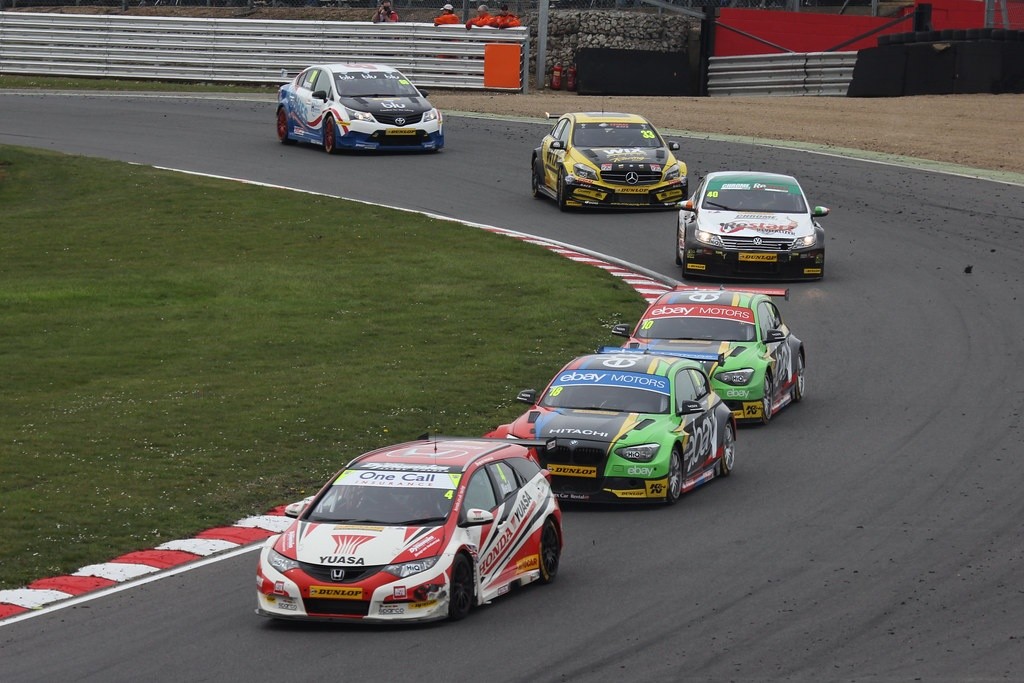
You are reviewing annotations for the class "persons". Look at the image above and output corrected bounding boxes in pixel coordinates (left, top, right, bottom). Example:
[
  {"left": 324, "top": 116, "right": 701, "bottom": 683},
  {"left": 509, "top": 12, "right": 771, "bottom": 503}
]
[
  {"left": 492, "top": 4, "right": 521, "bottom": 43},
  {"left": 466, "top": 5, "right": 496, "bottom": 75},
  {"left": 372, "top": 0, "right": 399, "bottom": 56},
  {"left": 433, "top": 4, "right": 460, "bottom": 73}
]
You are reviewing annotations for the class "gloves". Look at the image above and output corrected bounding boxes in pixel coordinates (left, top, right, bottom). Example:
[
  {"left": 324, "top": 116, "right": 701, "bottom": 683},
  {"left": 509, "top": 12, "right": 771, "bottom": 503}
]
[
  {"left": 498, "top": 24, "right": 504, "bottom": 30},
  {"left": 466, "top": 23, "right": 471, "bottom": 30}
]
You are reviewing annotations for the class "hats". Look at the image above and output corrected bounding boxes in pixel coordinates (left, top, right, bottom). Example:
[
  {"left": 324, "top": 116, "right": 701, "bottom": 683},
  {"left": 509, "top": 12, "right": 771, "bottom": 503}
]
[{"left": 440, "top": 4, "right": 453, "bottom": 10}]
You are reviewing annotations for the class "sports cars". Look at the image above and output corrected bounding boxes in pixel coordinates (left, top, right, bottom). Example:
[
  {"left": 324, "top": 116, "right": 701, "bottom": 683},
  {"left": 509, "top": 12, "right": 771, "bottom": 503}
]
[
  {"left": 531, "top": 112, "right": 691, "bottom": 210},
  {"left": 274, "top": 63, "right": 444, "bottom": 153},
  {"left": 255, "top": 433, "right": 563, "bottom": 626},
  {"left": 674, "top": 170, "right": 831, "bottom": 282},
  {"left": 484, "top": 347, "right": 737, "bottom": 506},
  {"left": 611, "top": 286, "right": 806, "bottom": 428}
]
